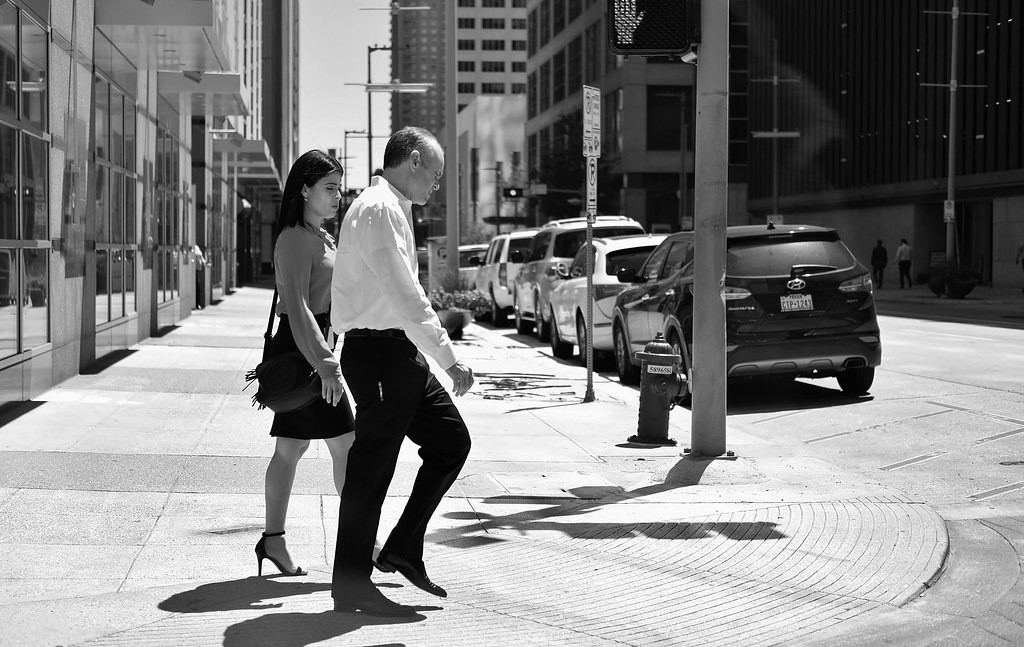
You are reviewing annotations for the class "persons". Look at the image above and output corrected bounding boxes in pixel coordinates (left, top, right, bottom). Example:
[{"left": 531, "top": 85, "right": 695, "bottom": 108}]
[
  {"left": 329, "top": 128, "right": 474, "bottom": 617},
  {"left": 254, "top": 150, "right": 356, "bottom": 580}
]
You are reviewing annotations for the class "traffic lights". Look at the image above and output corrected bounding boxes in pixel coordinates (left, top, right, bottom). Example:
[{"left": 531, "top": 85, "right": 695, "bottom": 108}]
[{"left": 606, "top": 0, "right": 696, "bottom": 60}]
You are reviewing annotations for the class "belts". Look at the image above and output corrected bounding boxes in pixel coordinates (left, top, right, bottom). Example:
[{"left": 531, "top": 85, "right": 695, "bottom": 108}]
[{"left": 345, "top": 328, "right": 410, "bottom": 341}]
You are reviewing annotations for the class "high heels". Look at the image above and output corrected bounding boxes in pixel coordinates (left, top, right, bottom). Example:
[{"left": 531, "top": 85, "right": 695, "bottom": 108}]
[{"left": 255, "top": 531, "right": 307, "bottom": 577}]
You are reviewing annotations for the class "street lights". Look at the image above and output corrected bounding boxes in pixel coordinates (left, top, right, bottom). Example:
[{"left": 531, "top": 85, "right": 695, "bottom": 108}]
[{"left": 340, "top": 2, "right": 437, "bottom": 234}]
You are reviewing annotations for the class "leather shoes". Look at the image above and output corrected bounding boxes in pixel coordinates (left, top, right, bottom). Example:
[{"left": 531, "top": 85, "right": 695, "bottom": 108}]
[
  {"left": 376, "top": 544, "right": 447, "bottom": 598},
  {"left": 334, "top": 586, "right": 417, "bottom": 617}
]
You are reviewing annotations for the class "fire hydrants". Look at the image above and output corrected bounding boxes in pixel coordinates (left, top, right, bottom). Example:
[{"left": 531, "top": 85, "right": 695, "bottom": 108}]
[{"left": 626, "top": 330, "right": 688, "bottom": 448}]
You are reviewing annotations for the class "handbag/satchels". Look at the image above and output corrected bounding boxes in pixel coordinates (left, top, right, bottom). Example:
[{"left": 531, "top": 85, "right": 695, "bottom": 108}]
[{"left": 241, "top": 332, "right": 334, "bottom": 414}]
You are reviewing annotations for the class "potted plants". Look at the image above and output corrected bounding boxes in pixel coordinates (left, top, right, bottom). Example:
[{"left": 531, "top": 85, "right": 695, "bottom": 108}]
[{"left": 938, "top": 256, "right": 981, "bottom": 298}]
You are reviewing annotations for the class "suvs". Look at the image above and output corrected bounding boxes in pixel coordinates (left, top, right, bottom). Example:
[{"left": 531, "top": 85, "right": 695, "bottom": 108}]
[
  {"left": 467, "top": 229, "right": 540, "bottom": 327},
  {"left": 611, "top": 222, "right": 881, "bottom": 404},
  {"left": 548, "top": 233, "right": 673, "bottom": 371},
  {"left": 512, "top": 215, "right": 646, "bottom": 342}
]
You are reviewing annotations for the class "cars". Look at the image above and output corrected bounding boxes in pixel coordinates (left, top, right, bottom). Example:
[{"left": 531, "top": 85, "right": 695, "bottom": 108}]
[{"left": 458, "top": 244, "right": 492, "bottom": 295}]
